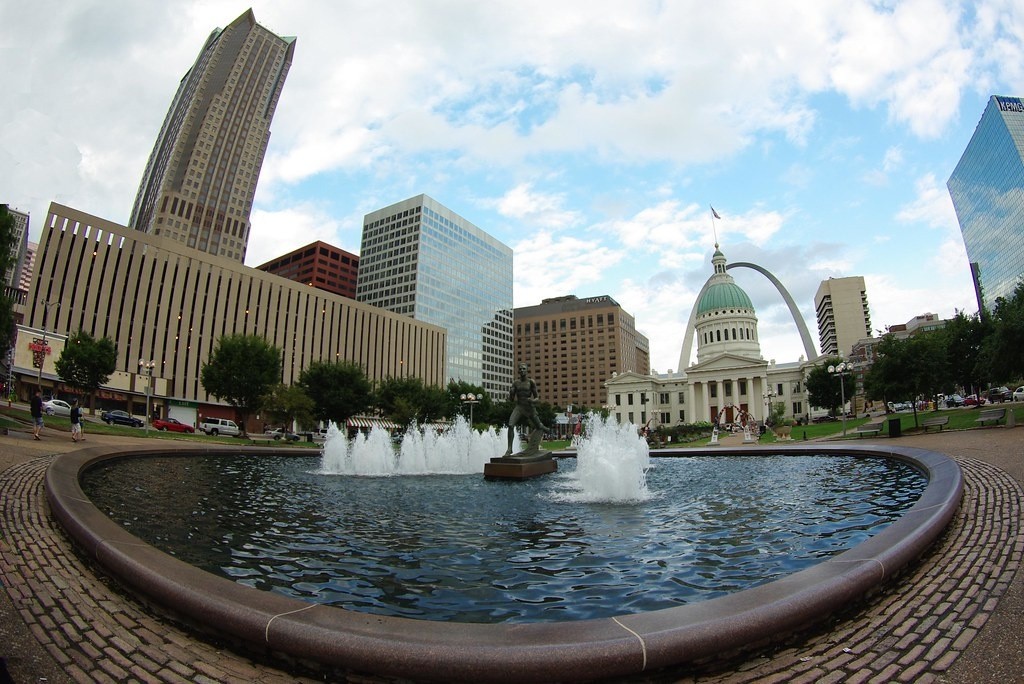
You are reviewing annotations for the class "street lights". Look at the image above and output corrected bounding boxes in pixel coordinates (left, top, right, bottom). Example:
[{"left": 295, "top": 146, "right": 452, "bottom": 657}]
[
  {"left": 38, "top": 298, "right": 60, "bottom": 396},
  {"left": 763, "top": 387, "right": 777, "bottom": 414},
  {"left": 828, "top": 363, "right": 853, "bottom": 435},
  {"left": 651, "top": 409, "right": 661, "bottom": 430},
  {"left": 139, "top": 358, "right": 157, "bottom": 433},
  {"left": 460, "top": 393, "right": 483, "bottom": 428}
]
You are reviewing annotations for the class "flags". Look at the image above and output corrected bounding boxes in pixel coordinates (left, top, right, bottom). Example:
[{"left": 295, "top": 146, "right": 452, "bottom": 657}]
[{"left": 710, "top": 205, "right": 720, "bottom": 218}]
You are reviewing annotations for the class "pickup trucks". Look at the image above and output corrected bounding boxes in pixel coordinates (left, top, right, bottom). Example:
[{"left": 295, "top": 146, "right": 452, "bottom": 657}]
[{"left": 199, "top": 417, "right": 239, "bottom": 436}]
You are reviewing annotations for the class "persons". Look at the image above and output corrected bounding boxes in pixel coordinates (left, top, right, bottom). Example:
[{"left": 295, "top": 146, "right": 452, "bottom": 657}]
[
  {"left": 640, "top": 426, "right": 658, "bottom": 446},
  {"left": 502, "top": 363, "right": 550, "bottom": 457},
  {"left": 30, "top": 388, "right": 45, "bottom": 441},
  {"left": 70, "top": 398, "right": 85, "bottom": 441},
  {"left": 282, "top": 423, "right": 286, "bottom": 439},
  {"left": 238, "top": 420, "right": 244, "bottom": 436}
]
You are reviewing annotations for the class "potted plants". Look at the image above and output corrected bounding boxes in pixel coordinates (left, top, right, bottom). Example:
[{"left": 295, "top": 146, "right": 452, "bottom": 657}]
[{"left": 767, "top": 401, "right": 795, "bottom": 440}]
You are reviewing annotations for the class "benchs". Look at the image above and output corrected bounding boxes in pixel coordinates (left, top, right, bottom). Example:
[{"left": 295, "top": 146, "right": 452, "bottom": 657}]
[
  {"left": 855, "top": 423, "right": 884, "bottom": 437},
  {"left": 249, "top": 434, "right": 275, "bottom": 445},
  {"left": 313, "top": 437, "right": 327, "bottom": 448},
  {"left": 974, "top": 408, "right": 1007, "bottom": 427},
  {"left": 921, "top": 416, "right": 951, "bottom": 431}
]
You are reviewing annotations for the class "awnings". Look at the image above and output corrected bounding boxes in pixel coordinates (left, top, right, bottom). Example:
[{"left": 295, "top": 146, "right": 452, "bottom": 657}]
[{"left": 346, "top": 416, "right": 450, "bottom": 430}]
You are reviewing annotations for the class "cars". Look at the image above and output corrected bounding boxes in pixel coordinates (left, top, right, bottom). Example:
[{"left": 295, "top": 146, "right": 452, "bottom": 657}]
[
  {"left": 42, "top": 398, "right": 81, "bottom": 417},
  {"left": 946, "top": 394, "right": 985, "bottom": 407},
  {"left": 153, "top": 417, "right": 194, "bottom": 433},
  {"left": 101, "top": 410, "right": 144, "bottom": 428},
  {"left": 265, "top": 428, "right": 301, "bottom": 441},
  {"left": 1012, "top": 386, "right": 1024, "bottom": 403}
]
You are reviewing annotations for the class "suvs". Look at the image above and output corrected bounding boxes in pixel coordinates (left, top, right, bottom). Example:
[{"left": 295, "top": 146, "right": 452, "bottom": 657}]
[{"left": 988, "top": 386, "right": 1013, "bottom": 404}]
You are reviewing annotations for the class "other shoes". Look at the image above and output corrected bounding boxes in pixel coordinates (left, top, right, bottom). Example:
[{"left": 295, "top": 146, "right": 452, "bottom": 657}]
[
  {"left": 81, "top": 438, "right": 86, "bottom": 440},
  {"left": 34, "top": 434, "right": 40, "bottom": 440},
  {"left": 72, "top": 436, "right": 77, "bottom": 442}
]
